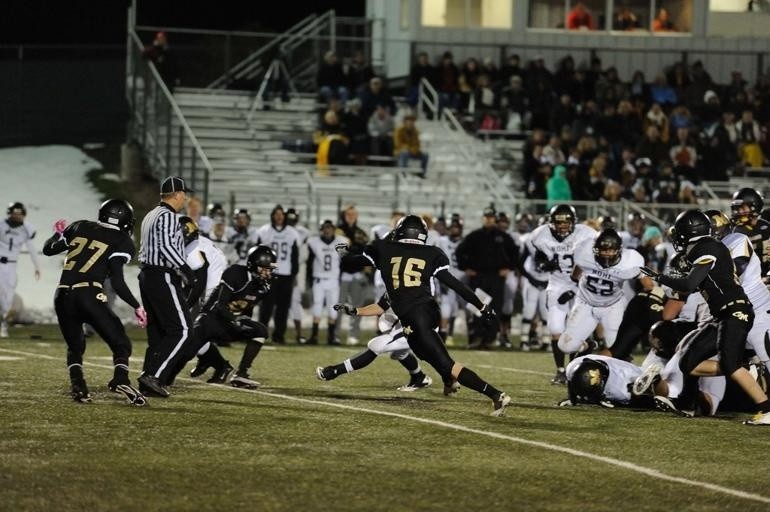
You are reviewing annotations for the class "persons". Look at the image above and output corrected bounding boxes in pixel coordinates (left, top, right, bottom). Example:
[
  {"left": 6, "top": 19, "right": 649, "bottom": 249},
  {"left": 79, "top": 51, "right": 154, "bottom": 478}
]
[
  {"left": 43, "top": 176, "right": 311, "bottom": 406},
  {"left": 567, "top": 2, "right": 678, "bottom": 33},
  {"left": 145, "top": 33, "right": 176, "bottom": 96},
  {"left": 0, "top": 203, "right": 40, "bottom": 340},
  {"left": 306, "top": 50, "right": 769, "bottom": 426}
]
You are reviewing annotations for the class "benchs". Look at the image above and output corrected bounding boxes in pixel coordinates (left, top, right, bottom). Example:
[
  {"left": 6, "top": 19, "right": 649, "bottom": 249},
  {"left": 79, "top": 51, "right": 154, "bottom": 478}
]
[{"left": 131, "top": 68, "right": 769, "bottom": 220}]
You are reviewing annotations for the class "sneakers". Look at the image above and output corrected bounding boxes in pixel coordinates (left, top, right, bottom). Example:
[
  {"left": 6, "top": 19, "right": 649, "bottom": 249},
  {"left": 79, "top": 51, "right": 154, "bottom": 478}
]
[
  {"left": 190, "top": 359, "right": 260, "bottom": 389},
  {"left": 746, "top": 410, "right": 770, "bottom": 426},
  {"left": 552, "top": 369, "right": 566, "bottom": 385},
  {"left": 489, "top": 391, "right": 511, "bottom": 416},
  {"left": 107, "top": 379, "right": 147, "bottom": 407},
  {"left": 443, "top": 381, "right": 460, "bottom": 395},
  {"left": 316, "top": 364, "right": 335, "bottom": 381},
  {"left": 633, "top": 361, "right": 661, "bottom": 396},
  {"left": 397, "top": 375, "right": 432, "bottom": 391},
  {"left": 72, "top": 379, "right": 93, "bottom": 404},
  {"left": 138, "top": 373, "right": 170, "bottom": 397},
  {"left": 654, "top": 395, "right": 696, "bottom": 418},
  {"left": 749, "top": 355, "right": 768, "bottom": 394}
]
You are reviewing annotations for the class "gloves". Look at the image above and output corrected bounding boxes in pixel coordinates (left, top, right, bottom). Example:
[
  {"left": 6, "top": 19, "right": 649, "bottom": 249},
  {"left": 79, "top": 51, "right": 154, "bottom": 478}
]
[
  {"left": 334, "top": 304, "right": 357, "bottom": 317},
  {"left": 481, "top": 304, "right": 496, "bottom": 321},
  {"left": 56, "top": 218, "right": 65, "bottom": 233},
  {"left": 639, "top": 266, "right": 663, "bottom": 287},
  {"left": 336, "top": 242, "right": 348, "bottom": 255},
  {"left": 135, "top": 308, "right": 148, "bottom": 327},
  {"left": 180, "top": 263, "right": 200, "bottom": 289}
]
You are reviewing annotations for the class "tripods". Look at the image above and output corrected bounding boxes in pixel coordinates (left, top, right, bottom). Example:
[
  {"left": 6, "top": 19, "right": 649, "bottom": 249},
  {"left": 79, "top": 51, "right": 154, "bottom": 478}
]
[{"left": 246, "top": 57, "right": 305, "bottom": 123}]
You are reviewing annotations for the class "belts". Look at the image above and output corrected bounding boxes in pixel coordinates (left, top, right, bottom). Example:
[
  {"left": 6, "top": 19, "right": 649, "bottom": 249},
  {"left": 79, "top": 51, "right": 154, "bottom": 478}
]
[
  {"left": 720, "top": 299, "right": 750, "bottom": 310},
  {"left": 58, "top": 282, "right": 102, "bottom": 289},
  {"left": 638, "top": 292, "right": 663, "bottom": 304}
]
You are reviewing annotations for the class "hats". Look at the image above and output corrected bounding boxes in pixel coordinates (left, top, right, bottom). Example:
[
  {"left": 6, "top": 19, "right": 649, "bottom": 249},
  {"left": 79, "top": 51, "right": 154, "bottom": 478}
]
[{"left": 161, "top": 175, "right": 195, "bottom": 194}]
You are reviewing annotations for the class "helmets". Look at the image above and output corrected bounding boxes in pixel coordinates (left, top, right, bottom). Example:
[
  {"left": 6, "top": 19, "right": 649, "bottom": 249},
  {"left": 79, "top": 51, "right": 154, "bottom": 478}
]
[
  {"left": 286, "top": 208, "right": 300, "bottom": 226},
  {"left": 99, "top": 199, "right": 136, "bottom": 227},
  {"left": 548, "top": 203, "right": 578, "bottom": 242},
  {"left": 591, "top": 229, "right": 623, "bottom": 268},
  {"left": 667, "top": 188, "right": 764, "bottom": 297},
  {"left": 247, "top": 245, "right": 278, "bottom": 280},
  {"left": 570, "top": 359, "right": 607, "bottom": 403},
  {"left": 179, "top": 216, "right": 199, "bottom": 247},
  {"left": 317, "top": 219, "right": 336, "bottom": 233},
  {"left": 483, "top": 206, "right": 547, "bottom": 231},
  {"left": 395, "top": 214, "right": 428, "bottom": 244},
  {"left": 7, "top": 202, "right": 26, "bottom": 216},
  {"left": 206, "top": 203, "right": 251, "bottom": 228}
]
